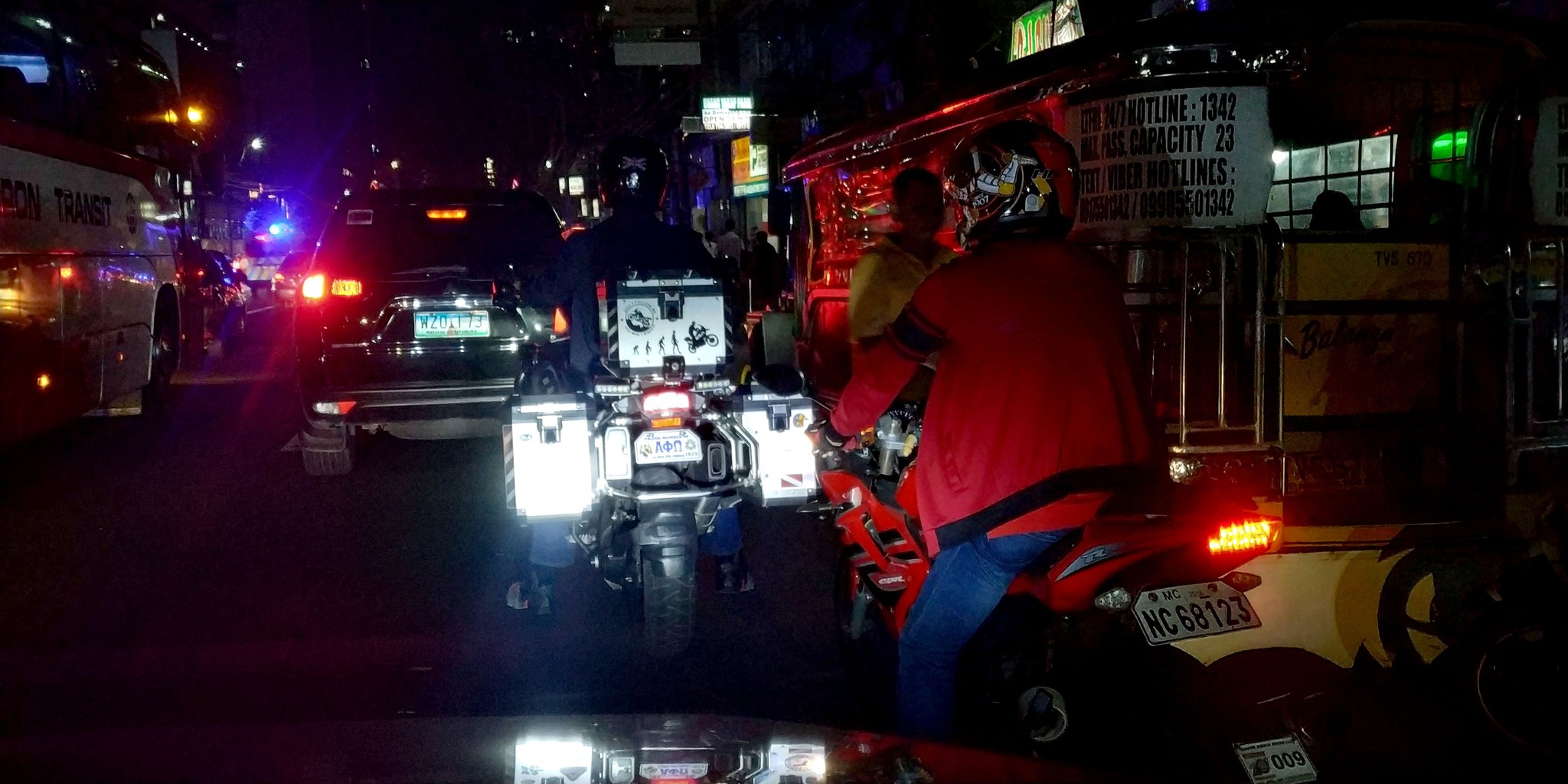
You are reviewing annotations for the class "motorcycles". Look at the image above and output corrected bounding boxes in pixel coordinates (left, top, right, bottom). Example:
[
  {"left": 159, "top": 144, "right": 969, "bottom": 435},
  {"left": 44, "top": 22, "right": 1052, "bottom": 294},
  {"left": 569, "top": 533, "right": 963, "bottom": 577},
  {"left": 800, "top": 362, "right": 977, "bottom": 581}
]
[
  {"left": 751, "top": 360, "right": 1323, "bottom": 784},
  {"left": 514, "top": 262, "right": 825, "bottom": 661}
]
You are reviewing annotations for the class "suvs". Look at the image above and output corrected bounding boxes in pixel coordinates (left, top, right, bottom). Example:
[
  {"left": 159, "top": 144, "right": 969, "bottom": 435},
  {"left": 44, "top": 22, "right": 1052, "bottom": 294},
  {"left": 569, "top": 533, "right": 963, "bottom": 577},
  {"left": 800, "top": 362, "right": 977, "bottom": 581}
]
[{"left": 274, "top": 177, "right": 590, "bottom": 478}]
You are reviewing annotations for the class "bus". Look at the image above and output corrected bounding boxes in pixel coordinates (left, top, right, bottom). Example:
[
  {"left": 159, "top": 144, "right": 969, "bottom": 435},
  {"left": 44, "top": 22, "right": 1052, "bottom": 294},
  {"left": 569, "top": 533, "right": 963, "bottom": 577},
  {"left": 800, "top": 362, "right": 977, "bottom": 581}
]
[
  {"left": 752, "top": 12, "right": 1566, "bottom": 782},
  {"left": 0, "top": 0, "right": 224, "bottom": 456}
]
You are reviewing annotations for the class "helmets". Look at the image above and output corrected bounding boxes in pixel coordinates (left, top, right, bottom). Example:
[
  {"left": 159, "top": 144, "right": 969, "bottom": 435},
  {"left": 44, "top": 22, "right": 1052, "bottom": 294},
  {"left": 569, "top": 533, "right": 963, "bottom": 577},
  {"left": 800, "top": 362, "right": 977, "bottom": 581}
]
[
  {"left": 941, "top": 119, "right": 1084, "bottom": 251},
  {"left": 596, "top": 145, "right": 670, "bottom": 217}
]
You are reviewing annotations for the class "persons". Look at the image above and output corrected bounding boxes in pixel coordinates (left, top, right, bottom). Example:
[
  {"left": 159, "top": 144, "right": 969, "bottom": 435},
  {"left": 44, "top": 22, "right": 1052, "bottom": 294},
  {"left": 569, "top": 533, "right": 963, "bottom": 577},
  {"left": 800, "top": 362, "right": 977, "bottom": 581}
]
[
  {"left": 817, "top": 119, "right": 1160, "bottom": 744},
  {"left": 501, "top": 138, "right": 755, "bottom": 621},
  {"left": 703, "top": 218, "right": 780, "bottom": 312}
]
